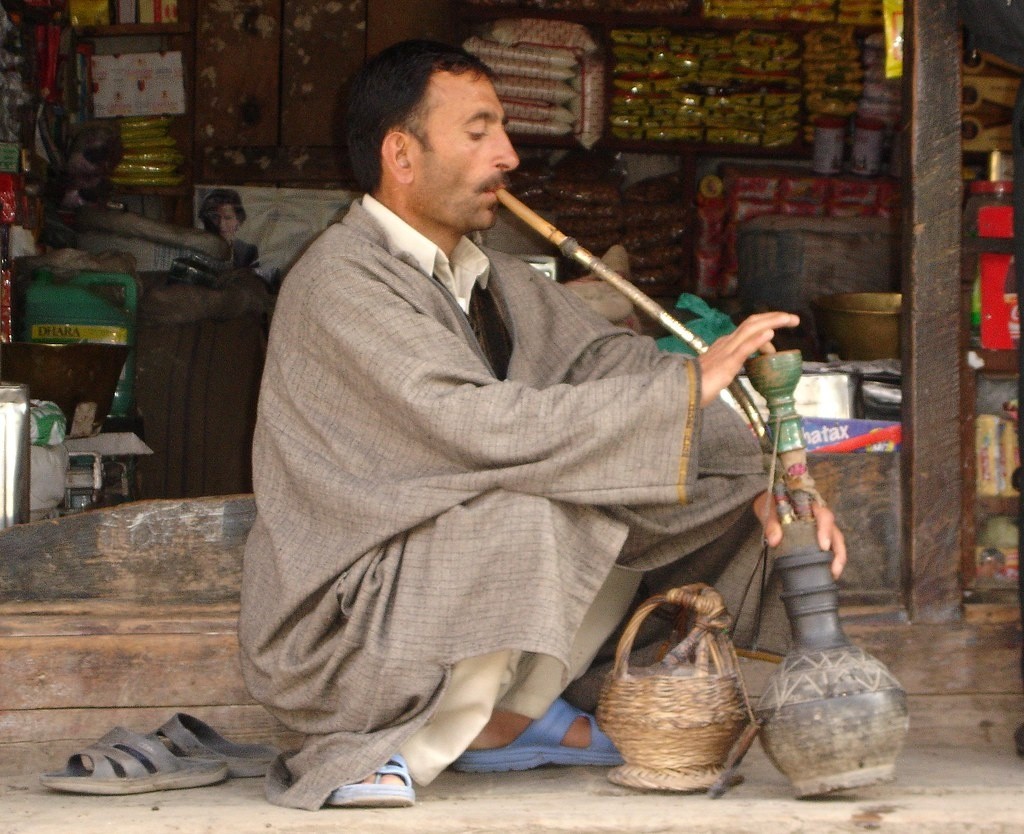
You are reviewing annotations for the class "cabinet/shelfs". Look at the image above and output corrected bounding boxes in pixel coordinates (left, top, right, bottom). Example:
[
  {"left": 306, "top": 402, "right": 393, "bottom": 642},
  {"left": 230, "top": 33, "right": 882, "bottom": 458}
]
[
  {"left": 0, "top": 0, "right": 367, "bottom": 347},
  {"left": 455, "top": 1, "right": 911, "bottom": 325}
]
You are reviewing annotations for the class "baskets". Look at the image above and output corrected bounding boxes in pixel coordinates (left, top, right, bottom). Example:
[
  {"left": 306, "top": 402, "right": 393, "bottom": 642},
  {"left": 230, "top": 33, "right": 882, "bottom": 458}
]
[{"left": 597, "top": 582, "right": 747, "bottom": 792}]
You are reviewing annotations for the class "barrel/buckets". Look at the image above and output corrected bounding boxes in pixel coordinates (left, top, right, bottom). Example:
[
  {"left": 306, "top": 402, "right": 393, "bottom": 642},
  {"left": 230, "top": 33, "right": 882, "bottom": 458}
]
[{"left": 24, "top": 269, "right": 137, "bottom": 415}]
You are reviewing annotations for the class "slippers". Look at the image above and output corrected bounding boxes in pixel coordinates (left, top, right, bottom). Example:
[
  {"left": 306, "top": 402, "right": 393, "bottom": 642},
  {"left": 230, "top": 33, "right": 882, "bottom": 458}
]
[
  {"left": 323, "top": 753, "right": 415, "bottom": 808},
  {"left": 142, "top": 713, "right": 284, "bottom": 778},
  {"left": 39, "top": 727, "right": 229, "bottom": 795},
  {"left": 450, "top": 695, "right": 626, "bottom": 773}
]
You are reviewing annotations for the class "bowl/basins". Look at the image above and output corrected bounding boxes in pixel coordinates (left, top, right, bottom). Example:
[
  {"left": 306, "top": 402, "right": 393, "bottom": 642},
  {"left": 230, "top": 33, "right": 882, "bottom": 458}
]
[{"left": 811, "top": 294, "right": 905, "bottom": 361}]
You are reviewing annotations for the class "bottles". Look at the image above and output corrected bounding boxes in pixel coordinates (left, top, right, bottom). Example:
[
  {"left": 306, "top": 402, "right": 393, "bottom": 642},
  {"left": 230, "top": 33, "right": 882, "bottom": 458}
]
[{"left": 64, "top": 455, "right": 95, "bottom": 513}]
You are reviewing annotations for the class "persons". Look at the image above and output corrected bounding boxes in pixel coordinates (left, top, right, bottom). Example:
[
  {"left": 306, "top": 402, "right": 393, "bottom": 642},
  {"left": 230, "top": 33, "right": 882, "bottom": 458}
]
[
  {"left": 238, "top": 36, "right": 848, "bottom": 808},
  {"left": 198, "top": 186, "right": 262, "bottom": 275}
]
